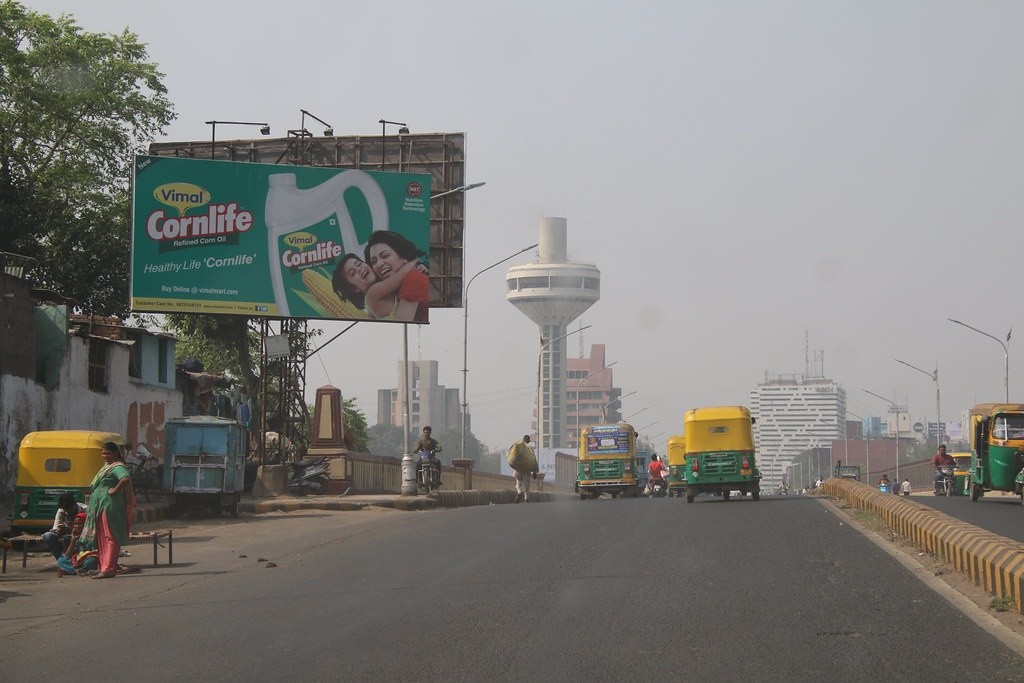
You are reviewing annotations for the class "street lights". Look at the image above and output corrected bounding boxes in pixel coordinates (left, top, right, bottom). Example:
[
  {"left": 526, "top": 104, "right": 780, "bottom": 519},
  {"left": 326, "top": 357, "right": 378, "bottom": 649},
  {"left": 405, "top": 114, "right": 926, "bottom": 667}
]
[
  {"left": 894, "top": 358, "right": 940, "bottom": 454},
  {"left": 806, "top": 409, "right": 870, "bottom": 486},
  {"left": 400, "top": 182, "right": 487, "bottom": 495},
  {"left": 861, "top": 388, "right": 900, "bottom": 483},
  {"left": 576, "top": 361, "right": 637, "bottom": 471},
  {"left": 451, "top": 244, "right": 539, "bottom": 468},
  {"left": 532, "top": 324, "right": 592, "bottom": 479},
  {"left": 947, "top": 317, "right": 1012, "bottom": 405}
]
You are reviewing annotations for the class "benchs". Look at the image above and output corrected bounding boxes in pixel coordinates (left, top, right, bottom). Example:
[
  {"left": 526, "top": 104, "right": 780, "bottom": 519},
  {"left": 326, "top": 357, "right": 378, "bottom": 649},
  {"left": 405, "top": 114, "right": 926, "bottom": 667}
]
[{"left": 2, "top": 529, "right": 172, "bottom": 574}]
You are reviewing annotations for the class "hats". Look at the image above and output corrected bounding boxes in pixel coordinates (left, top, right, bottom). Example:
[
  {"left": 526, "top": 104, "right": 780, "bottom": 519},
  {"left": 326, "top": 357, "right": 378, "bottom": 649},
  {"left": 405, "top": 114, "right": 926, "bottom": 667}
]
[{"left": 74, "top": 513, "right": 87, "bottom": 524}]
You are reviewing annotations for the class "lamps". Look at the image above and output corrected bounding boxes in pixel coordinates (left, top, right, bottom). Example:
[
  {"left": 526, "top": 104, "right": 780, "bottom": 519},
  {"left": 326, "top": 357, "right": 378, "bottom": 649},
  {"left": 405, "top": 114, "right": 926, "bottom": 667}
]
[
  {"left": 206, "top": 121, "right": 269, "bottom": 160},
  {"left": 379, "top": 120, "right": 409, "bottom": 171},
  {"left": 300, "top": 109, "right": 333, "bottom": 164}
]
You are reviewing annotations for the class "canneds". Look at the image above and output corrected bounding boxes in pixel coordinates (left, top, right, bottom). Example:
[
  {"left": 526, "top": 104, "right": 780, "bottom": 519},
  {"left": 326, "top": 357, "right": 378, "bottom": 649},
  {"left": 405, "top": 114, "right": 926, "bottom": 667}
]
[{"left": 57, "top": 569, "right": 63, "bottom": 578}]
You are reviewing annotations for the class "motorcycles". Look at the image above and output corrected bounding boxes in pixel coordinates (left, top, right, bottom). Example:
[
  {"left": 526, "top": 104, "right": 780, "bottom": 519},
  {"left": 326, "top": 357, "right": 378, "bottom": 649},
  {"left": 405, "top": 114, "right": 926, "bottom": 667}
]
[
  {"left": 650, "top": 477, "right": 663, "bottom": 497},
  {"left": 9, "top": 431, "right": 126, "bottom": 550},
  {"left": 966, "top": 403, "right": 1024, "bottom": 505},
  {"left": 683, "top": 406, "right": 763, "bottom": 502},
  {"left": 667, "top": 436, "right": 722, "bottom": 497},
  {"left": 413, "top": 449, "right": 440, "bottom": 495},
  {"left": 947, "top": 452, "right": 984, "bottom": 497},
  {"left": 933, "top": 464, "right": 959, "bottom": 496}
]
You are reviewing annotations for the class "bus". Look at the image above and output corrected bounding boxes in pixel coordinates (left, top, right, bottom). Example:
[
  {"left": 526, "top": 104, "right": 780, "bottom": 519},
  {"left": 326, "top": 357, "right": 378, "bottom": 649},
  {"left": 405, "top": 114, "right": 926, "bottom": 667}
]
[{"left": 576, "top": 423, "right": 639, "bottom": 499}]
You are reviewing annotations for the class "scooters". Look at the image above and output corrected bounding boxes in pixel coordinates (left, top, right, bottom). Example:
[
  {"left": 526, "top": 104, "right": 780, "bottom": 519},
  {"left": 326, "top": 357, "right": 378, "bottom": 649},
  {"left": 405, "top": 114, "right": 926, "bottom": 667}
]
[{"left": 266, "top": 437, "right": 333, "bottom": 495}]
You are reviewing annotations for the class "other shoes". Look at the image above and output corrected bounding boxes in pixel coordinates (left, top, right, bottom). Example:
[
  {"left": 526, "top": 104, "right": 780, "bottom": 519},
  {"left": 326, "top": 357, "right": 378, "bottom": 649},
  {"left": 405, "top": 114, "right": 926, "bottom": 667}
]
[
  {"left": 92, "top": 571, "right": 115, "bottom": 581},
  {"left": 76, "top": 566, "right": 88, "bottom": 573},
  {"left": 118, "top": 549, "right": 130, "bottom": 557},
  {"left": 415, "top": 477, "right": 423, "bottom": 484},
  {"left": 437, "top": 480, "right": 442, "bottom": 485},
  {"left": 936, "top": 488, "right": 940, "bottom": 495},
  {"left": 515, "top": 494, "right": 522, "bottom": 503},
  {"left": 524, "top": 499, "right": 530, "bottom": 503}
]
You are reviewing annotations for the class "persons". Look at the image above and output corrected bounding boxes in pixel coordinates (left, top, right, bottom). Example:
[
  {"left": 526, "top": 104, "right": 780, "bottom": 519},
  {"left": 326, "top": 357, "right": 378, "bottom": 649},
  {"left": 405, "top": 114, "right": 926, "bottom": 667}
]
[
  {"left": 78, "top": 442, "right": 137, "bottom": 579},
  {"left": 511, "top": 435, "right": 536, "bottom": 504},
  {"left": 646, "top": 453, "right": 667, "bottom": 496},
  {"left": 930, "top": 444, "right": 957, "bottom": 495},
  {"left": 802, "top": 476, "right": 824, "bottom": 493},
  {"left": 57, "top": 512, "right": 98, "bottom": 576},
  {"left": 778, "top": 481, "right": 789, "bottom": 495},
  {"left": 364, "top": 230, "right": 429, "bottom": 309},
  {"left": 43, "top": 493, "right": 88, "bottom": 573},
  {"left": 892, "top": 479, "right": 901, "bottom": 496},
  {"left": 332, "top": 253, "right": 429, "bottom": 321},
  {"left": 902, "top": 478, "right": 912, "bottom": 495},
  {"left": 879, "top": 474, "right": 891, "bottom": 484},
  {"left": 411, "top": 425, "right": 443, "bottom": 487}
]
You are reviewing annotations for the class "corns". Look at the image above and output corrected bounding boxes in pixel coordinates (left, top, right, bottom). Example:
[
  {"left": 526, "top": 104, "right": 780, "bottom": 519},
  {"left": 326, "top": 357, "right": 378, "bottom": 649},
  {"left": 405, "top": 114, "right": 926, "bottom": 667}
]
[{"left": 301, "top": 269, "right": 367, "bottom": 319}]
